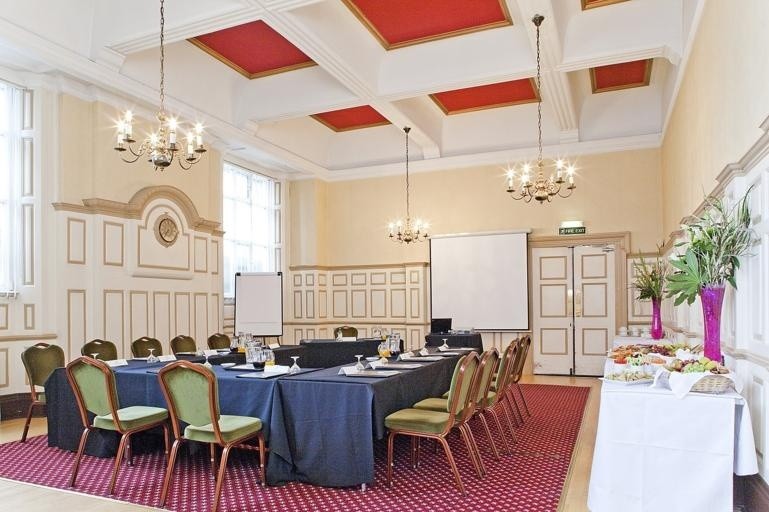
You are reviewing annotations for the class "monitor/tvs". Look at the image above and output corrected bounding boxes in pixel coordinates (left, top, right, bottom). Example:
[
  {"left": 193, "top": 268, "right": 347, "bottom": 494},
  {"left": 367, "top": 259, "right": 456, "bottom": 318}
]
[{"left": 430, "top": 318, "right": 453, "bottom": 335}]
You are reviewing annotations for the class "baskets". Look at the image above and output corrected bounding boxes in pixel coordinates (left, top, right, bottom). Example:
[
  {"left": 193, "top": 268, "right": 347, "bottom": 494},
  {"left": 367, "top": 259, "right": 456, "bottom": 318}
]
[{"left": 690, "top": 375, "right": 732, "bottom": 394}]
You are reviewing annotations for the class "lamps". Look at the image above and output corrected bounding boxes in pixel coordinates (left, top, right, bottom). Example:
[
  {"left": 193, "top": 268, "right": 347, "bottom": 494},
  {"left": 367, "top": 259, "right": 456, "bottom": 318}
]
[
  {"left": 502, "top": 14, "right": 576, "bottom": 202},
  {"left": 385, "top": 127, "right": 434, "bottom": 243},
  {"left": 113, "top": 0, "right": 211, "bottom": 170}
]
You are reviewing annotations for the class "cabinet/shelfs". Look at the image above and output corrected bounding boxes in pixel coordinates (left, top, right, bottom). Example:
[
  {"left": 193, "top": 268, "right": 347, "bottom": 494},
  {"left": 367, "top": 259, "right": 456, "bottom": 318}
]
[{"left": 528, "top": 231, "right": 632, "bottom": 377}]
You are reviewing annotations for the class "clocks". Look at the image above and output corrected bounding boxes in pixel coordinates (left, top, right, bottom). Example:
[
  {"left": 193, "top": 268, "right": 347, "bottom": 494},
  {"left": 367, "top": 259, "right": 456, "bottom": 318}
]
[{"left": 153, "top": 212, "right": 180, "bottom": 247}]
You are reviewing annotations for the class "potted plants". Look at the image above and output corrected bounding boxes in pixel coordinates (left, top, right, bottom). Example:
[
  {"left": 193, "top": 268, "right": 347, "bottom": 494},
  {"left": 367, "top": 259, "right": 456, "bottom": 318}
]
[
  {"left": 631, "top": 243, "right": 669, "bottom": 339},
  {"left": 666, "top": 182, "right": 753, "bottom": 362}
]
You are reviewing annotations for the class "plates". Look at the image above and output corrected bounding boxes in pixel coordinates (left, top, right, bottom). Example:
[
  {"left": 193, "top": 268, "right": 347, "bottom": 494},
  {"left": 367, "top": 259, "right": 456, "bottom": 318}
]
[{"left": 597, "top": 346, "right": 676, "bottom": 386}]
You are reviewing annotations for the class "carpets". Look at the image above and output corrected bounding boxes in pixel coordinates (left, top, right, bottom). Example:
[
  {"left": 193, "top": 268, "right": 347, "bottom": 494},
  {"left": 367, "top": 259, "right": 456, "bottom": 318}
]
[{"left": 1, "top": 384, "right": 592, "bottom": 512}]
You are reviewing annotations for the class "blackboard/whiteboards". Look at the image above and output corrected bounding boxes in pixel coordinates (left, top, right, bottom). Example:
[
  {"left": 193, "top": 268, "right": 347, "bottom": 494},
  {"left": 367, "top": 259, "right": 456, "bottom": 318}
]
[{"left": 234, "top": 272, "right": 282, "bottom": 337}]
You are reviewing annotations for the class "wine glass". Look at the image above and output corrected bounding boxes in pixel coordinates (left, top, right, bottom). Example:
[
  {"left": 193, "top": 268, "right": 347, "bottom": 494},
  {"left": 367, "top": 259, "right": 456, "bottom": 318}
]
[{"left": 85, "top": 326, "right": 453, "bottom": 374}]
